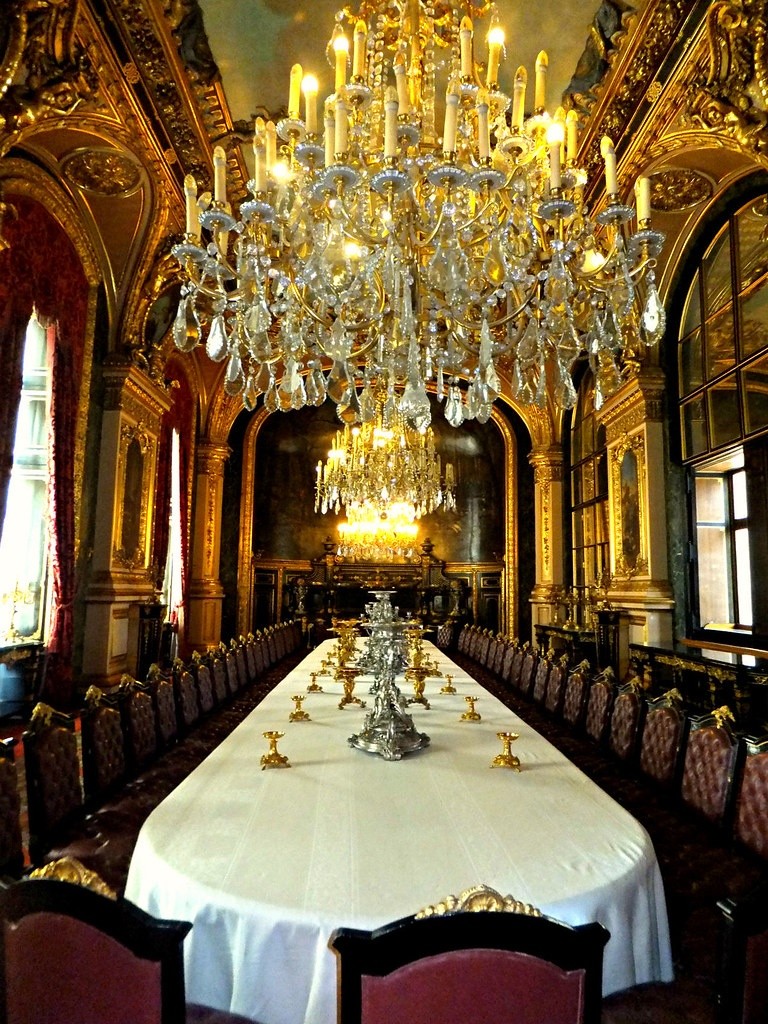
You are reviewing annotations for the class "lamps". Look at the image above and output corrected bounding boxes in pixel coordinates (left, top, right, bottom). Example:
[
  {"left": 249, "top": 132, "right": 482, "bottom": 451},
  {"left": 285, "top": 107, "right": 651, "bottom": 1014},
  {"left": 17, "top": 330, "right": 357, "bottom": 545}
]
[{"left": 170, "top": 0, "right": 670, "bottom": 559}]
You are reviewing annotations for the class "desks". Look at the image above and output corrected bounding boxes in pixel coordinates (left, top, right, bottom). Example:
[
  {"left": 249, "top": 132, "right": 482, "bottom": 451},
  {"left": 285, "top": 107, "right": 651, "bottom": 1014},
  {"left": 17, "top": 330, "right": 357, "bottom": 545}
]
[{"left": 122, "top": 636, "right": 674, "bottom": 1024}]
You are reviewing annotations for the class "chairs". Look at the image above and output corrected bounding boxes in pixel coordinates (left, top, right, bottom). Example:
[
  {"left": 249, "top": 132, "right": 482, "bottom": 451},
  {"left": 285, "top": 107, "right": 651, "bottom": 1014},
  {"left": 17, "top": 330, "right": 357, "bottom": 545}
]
[
  {"left": 1, "top": 857, "right": 194, "bottom": 1024},
  {"left": 1, "top": 618, "right": 322, "bottom": 896},
  {"left": 433, "top": 621, "right": 768, "bottom": 1023},
  {"left": 327, "top": 885, "right": 613, "bottom": 1024}
]
